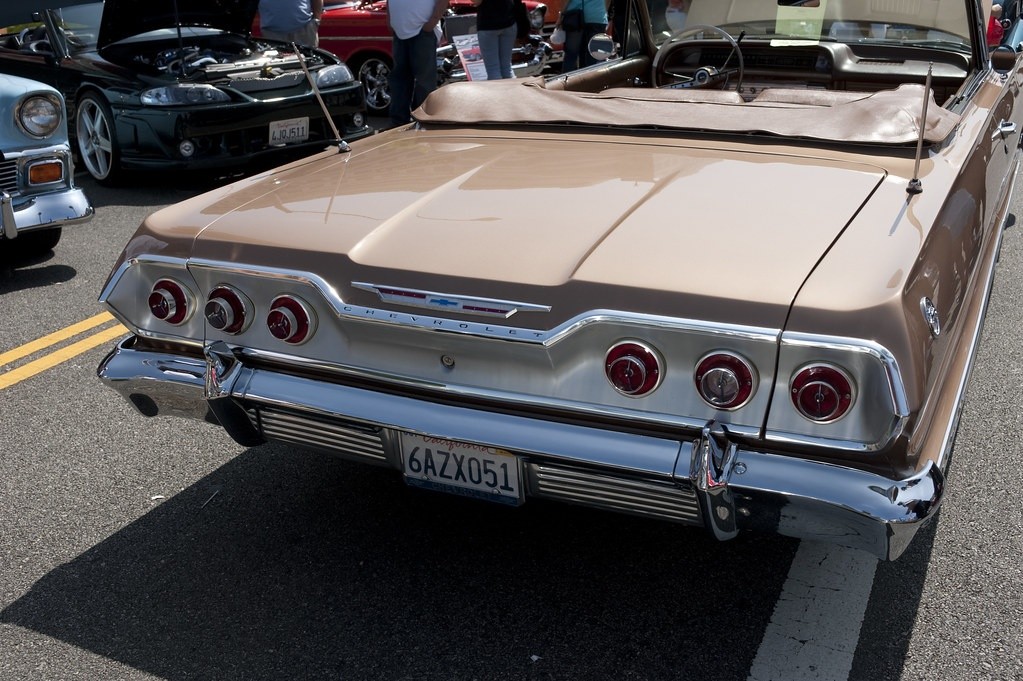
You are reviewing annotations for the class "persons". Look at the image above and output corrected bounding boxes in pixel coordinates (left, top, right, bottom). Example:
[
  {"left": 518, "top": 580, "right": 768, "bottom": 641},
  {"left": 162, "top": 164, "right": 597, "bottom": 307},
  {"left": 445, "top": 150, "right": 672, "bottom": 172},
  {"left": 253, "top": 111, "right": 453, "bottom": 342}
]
[
  {"left": 473, "top": 0, "right": 521, "bottom": 80},
  {"left": 258, "top": 0, "right": 323, "bottom": 49},
  {"left": 987, "top": 0, "right": 1004, "bottom": 52},
  {"left": 379, "top": 0, "right": 449, "bottom": 132},
  {"left": 555, "top": 0, "right": 639, "bottom": 73}
]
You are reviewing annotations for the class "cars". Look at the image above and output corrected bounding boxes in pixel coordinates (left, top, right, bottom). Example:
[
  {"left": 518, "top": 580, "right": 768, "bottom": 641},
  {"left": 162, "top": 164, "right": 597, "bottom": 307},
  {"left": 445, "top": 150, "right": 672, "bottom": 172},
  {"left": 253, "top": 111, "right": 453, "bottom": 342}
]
[
  {"left": 0, "top": 0, "right": 587, "bottom": 251},
  {"left": 94, "top": 0, "right": 1021, "bottom": 564}
]
